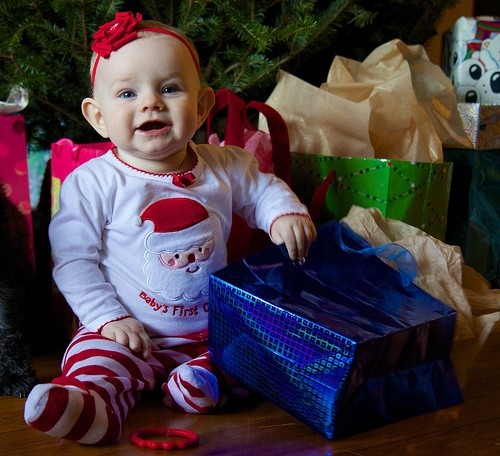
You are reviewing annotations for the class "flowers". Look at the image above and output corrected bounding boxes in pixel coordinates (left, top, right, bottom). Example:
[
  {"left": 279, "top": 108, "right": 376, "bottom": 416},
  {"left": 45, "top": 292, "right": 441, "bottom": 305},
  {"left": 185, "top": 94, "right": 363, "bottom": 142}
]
[{"left": 90, "top": 11, "right": 143, "bottom": 59}]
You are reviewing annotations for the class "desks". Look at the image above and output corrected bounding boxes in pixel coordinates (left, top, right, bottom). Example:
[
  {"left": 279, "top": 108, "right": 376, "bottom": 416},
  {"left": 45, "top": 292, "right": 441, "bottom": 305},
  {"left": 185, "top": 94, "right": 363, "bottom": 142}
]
[{"left": 0, "top": 390, "right": 500, "bottom": 456}]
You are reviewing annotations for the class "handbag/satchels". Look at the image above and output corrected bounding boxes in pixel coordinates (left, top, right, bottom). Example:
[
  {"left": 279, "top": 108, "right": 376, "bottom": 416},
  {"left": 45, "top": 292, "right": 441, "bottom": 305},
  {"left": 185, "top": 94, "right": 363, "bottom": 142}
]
[
  {"left": 0, "top": 39, "right": 500, "bottom": 358},
  {"left": 208, "top": 221, "right": 464, "bottom": 442}
]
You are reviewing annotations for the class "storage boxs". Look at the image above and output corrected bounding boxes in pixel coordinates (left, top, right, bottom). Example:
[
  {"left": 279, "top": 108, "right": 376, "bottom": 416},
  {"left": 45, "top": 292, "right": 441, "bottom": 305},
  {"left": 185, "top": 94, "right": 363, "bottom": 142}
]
[
  {"left": 455, "top": 100, "right": 499, "bottom": 150},
  {"left": 209, "top": 235, "right": 465, "bottom": 440}
]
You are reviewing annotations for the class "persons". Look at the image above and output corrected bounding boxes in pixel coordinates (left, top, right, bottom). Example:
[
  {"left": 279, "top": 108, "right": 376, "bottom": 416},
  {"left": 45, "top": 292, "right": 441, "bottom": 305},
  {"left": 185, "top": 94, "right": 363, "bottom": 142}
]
[{"left": 24, "top": 12, "right": 318, "bottom": 446}]
